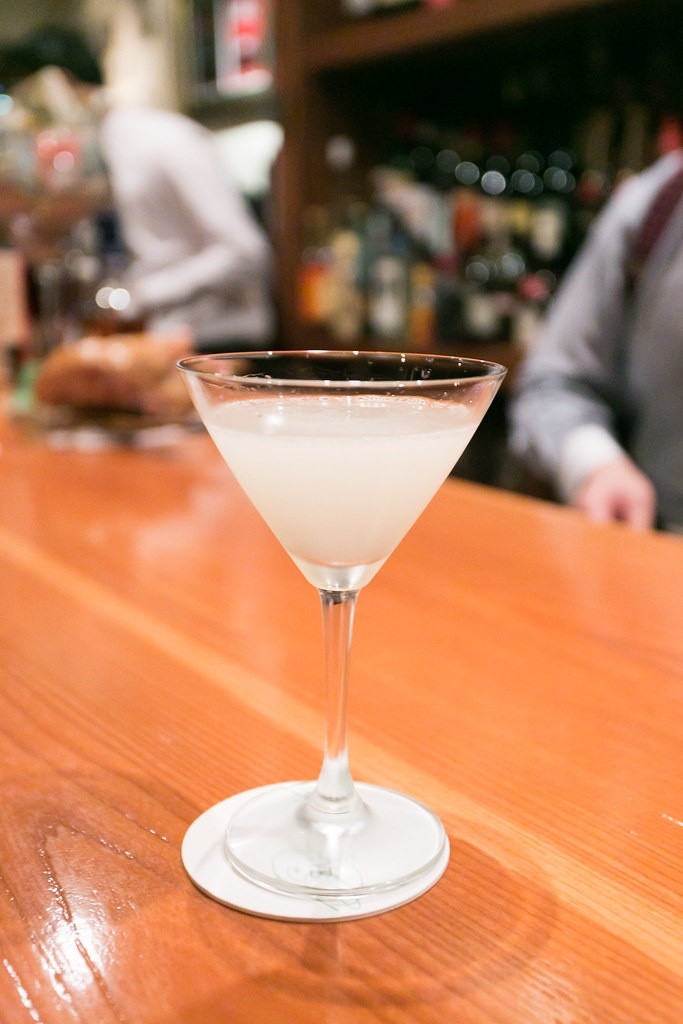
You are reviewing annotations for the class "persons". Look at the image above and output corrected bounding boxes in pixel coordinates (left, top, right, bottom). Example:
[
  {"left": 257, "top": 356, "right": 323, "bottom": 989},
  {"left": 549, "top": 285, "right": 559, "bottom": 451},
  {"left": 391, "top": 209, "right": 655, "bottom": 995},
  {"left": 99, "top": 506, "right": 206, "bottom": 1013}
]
[
  {"left": 507, "top": 149, "right": 683, "bottom": 534},
  {"left": 2, "top": 19, "right": 275, "bottom": 358}
]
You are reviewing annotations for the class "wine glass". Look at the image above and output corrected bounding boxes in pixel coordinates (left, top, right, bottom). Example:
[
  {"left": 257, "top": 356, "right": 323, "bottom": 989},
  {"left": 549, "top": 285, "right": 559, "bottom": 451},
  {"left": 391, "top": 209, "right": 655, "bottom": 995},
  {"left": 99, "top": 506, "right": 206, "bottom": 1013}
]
[{"left": 180, "top": 351, "right": 503, "bottom": 894}]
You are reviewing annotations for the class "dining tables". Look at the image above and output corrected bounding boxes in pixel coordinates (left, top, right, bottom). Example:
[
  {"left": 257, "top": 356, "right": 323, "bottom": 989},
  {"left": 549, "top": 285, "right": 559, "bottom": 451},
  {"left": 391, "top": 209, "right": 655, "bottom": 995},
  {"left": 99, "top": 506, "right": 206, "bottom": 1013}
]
[{"left": 0, "top": 395, "right": 683, "bottom": 1024}]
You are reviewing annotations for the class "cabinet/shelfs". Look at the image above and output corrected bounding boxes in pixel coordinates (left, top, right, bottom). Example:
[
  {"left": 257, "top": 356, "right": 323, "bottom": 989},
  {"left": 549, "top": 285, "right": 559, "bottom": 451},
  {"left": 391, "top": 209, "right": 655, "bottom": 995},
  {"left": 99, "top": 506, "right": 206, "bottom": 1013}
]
[{"left": 271, "top": 1, "right": 683, "bottom": 505}]
